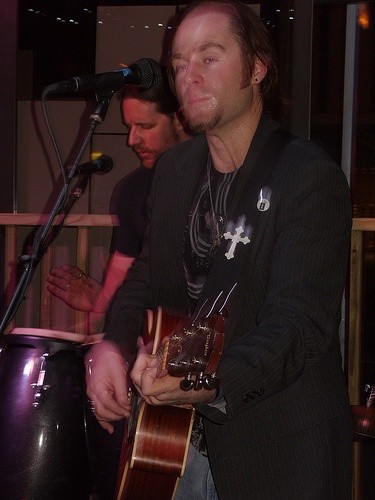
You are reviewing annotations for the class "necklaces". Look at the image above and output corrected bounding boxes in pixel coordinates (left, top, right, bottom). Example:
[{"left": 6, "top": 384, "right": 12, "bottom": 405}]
[{"left": 206, "top": 159, "right": 237, "bottom": 251}]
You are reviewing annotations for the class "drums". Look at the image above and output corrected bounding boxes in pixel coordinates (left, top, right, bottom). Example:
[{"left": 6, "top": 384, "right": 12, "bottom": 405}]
[{"left": 0, "top": 327, "right": 115, "bottom": 499}]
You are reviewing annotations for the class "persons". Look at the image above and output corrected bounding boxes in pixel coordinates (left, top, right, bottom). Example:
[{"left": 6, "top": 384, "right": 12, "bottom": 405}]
[
  {"left": 47, "top": 69, "right": 199, "bottom": 314},
  {"left": 81, "top": 0, "right": 353, "bottom": 500}
]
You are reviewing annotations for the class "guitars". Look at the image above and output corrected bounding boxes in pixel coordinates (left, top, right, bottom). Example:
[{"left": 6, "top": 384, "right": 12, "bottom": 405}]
[{"left": 116, "top": 163, "right": 293, "bottom": 500}]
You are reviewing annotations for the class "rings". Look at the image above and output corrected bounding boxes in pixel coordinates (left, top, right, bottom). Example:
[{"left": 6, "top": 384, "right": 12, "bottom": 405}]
[
  {"left": 90, "top": 402, "right": 95, "bottom": 413},
  {"left": 63, "top": 284, "right": 70, "bottom": 290},
  {"left": 79, "top": 272, "right": 84, "bottom": 279}
]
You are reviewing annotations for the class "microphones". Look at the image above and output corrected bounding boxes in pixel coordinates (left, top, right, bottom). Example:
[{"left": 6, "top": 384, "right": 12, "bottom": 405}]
[
  {"left": 73, "top": 155, "right": 115, "bottom": 176},
  {"left": 45, "top": 58, "right": 161, "bottom": 94}
]
